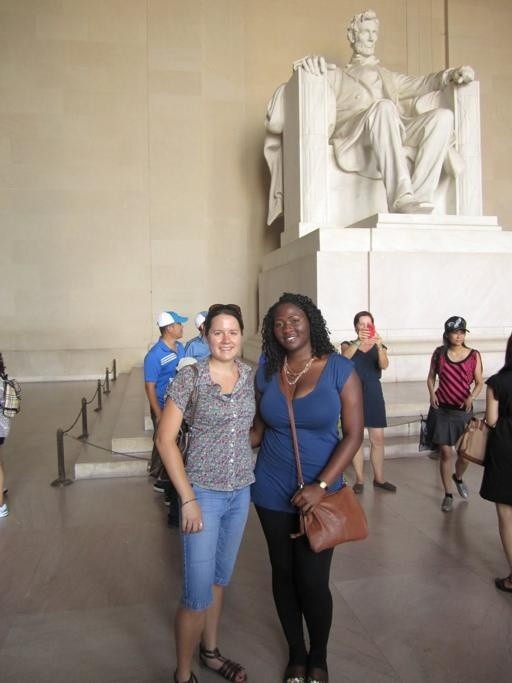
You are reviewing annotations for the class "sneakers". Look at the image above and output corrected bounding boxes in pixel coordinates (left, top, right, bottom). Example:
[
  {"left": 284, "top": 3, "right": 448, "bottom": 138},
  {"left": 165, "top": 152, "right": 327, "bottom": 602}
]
[
  {"left": 353, "top": 484, "right": 363, "bottom": 493},
  {"left": 373, "top": 480, "right": 395, "bottom": 491},
  {"left": 442, "top": 496, "right": 453, "bottom": 512},
  {"left": 452, "top": 474, "right": 468, "bottom": 498}
]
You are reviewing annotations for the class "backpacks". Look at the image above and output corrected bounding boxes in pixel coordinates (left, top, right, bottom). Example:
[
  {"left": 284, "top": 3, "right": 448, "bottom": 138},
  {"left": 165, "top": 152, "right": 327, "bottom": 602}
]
[{"left": 1, "top": 372, "right": 22, "bottom": 418}]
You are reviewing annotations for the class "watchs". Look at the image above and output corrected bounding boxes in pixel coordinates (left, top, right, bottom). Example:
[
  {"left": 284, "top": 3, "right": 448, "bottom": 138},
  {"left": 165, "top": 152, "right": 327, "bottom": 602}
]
[{"left": 314, "top": 478, "right": 329, "bottom": 491}]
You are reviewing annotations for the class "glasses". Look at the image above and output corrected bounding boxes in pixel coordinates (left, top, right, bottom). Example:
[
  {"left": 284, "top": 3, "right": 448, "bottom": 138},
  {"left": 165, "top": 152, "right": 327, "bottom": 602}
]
[{"left": 209, "top": 304, "right": 240, "bottom": 313}]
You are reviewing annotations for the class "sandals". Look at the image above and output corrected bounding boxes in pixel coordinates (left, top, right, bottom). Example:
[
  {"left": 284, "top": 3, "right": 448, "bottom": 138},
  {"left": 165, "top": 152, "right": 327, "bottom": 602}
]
[
  {"left": 199, "top": 642, "right": 247, "bottom": 683},
  {"left": 495, "top": 575, "right": 512, "bottom": 592}
]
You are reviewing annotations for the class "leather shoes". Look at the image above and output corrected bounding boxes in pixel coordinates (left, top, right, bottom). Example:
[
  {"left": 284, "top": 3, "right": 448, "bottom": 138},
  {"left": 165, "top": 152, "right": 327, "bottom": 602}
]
[
  {"left": 285, "top": 659, "right": 307, "bottom": 683},
  {"left": 306, "top": 664, "right": 328, "bottom": 683}
]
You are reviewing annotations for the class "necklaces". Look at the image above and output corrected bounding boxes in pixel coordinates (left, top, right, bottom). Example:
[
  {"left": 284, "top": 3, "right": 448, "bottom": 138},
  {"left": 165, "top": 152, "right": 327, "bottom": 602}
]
[{"left": 284, "top": 354, "right": 315, "bottom": 385}]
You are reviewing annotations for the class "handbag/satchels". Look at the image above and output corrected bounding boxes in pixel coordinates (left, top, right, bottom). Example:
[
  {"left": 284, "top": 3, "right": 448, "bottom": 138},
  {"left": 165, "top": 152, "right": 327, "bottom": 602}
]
[
  {"left": 418, "top": 415, "right": 437, "bottom": 451},
  {"left": 290, "top": 482, "right": 369, "bottom": 553},
  {"left": 148, "top": 421, "right": 189, "bottom": 482},
  {"left": 457, "top": 416, "right": 489, "bottom": 465}
]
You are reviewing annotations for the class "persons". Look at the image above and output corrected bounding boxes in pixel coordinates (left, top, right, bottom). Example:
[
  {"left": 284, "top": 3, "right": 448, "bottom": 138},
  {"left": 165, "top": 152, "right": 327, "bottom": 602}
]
[
  {"left": 145, "top": 310, "right": 211, "bottom": 528},
  {"left": 419, "top": 315, "right": 484, "bottom": 512},
  {"left": 0, "top": 351, "right": 24, "bottom": 520},
  {"left": 455, "top": 330, "right": 512, "bottom": 594},
  {"left": 249, "top": 292, "right": 363, "bottom": 683},
  {"left": 340, "top": 310, "right": 398, "bottom": 494},
  {"left": 153, "top": 303, "right": 257, "bottom": 683},
  {"left": 262, "top": 8, "right": 477, "bottom": 228}
]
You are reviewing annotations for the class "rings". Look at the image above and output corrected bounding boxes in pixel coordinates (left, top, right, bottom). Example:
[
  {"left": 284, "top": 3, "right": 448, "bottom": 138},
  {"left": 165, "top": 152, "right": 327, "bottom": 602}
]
[{"left": 200, "top": 522, "right": 204, "bottom": 527}]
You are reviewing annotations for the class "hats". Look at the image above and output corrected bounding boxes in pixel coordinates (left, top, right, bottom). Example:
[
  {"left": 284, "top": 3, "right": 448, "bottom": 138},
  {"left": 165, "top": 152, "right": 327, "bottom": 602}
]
[
  {"left": 445, "top": 316, "right": 470, "bottom": 333},
  {"left": 156, "top": 311, "right": 208, "bottom": 371}
]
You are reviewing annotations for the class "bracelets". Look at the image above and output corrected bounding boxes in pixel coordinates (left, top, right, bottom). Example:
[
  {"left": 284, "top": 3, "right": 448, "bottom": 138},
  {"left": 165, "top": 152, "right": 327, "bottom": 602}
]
[
  {"left": 182, "top": 499, "right": 198, "bottom": 506},
  {"left": 354, "top": 342, "right": 359, "bottom": 347},
  {"left": 470, "top": 394, "right": 476, "bottom": 401}
]
